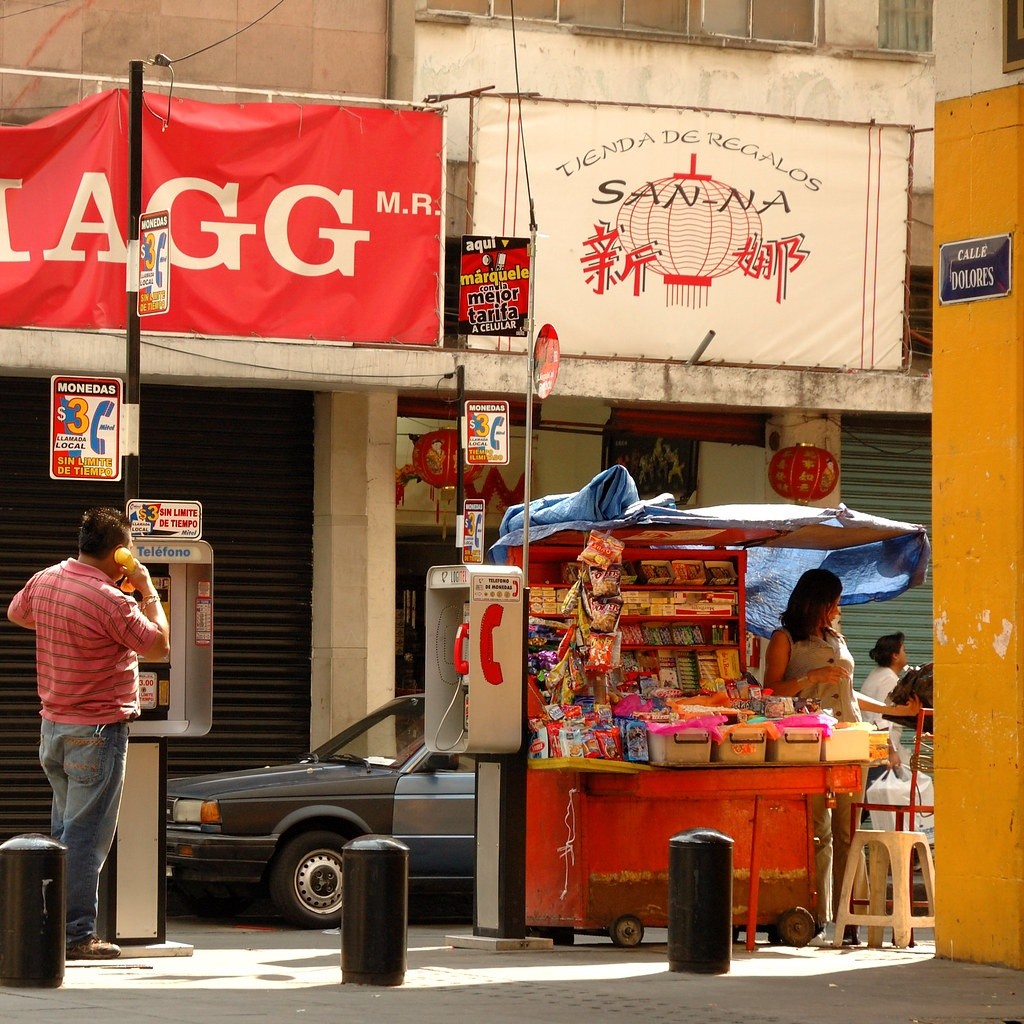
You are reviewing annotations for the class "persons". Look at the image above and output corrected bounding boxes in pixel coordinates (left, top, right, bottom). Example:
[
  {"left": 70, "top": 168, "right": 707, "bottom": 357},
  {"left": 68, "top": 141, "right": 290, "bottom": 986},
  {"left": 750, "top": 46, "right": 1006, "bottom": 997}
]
[
  {"left": 7, "top": 505, "right": 171, "bottom": 961},
  {"left": 762, "top": 568, "right": 869, "bottom": 946},
  {"left": 860, "top": 630, "right": 922, "bottom": 871}
]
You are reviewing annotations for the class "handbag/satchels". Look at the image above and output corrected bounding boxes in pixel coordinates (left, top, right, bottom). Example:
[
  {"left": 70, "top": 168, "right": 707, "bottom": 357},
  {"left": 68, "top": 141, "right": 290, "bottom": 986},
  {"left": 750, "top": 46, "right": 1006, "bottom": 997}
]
[{"left": 866, "top": 763, "right": 934, "bottom": 848}]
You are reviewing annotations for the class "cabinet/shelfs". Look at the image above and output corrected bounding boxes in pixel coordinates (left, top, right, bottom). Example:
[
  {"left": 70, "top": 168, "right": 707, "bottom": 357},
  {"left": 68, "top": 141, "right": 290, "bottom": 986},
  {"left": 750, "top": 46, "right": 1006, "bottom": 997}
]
[{"left": 510, "top": 549, "right": 748, "bottom": 716}]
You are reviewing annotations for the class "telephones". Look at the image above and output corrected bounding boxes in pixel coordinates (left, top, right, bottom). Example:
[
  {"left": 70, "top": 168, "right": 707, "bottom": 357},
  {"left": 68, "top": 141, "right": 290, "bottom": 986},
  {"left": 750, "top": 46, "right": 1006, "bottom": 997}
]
[
  {"left": 111, "top": 547, "right": 137, "bottom": 594},
  {"left": 454, "top": 601, "right": 469, "bottom": 687}
]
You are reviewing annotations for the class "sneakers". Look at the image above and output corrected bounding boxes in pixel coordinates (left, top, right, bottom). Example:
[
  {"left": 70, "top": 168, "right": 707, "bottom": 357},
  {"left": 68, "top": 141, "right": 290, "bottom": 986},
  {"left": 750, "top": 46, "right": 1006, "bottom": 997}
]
[
  {"left": 809, "top": 927, "right": 826, "bottom": 947},
  {"left": 842, "top": 929, "right": 861, "bottom": 945},
  {"left": 66, "top": 936, "right": 121, "bottom": 959}
]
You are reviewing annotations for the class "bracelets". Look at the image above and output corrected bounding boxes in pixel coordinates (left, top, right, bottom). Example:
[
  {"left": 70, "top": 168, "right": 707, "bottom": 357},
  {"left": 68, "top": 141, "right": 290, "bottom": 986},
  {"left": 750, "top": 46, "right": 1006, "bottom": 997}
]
[
  {"left": 797, "top": 670, "right": 816, "bottom": 691},
  {"left": 142, "top": 595, "right": 160, "bottom": 610}
]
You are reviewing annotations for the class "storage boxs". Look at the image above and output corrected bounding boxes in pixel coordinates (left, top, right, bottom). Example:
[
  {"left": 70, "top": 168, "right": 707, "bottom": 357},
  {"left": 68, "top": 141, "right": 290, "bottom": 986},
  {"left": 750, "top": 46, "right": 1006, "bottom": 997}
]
[{"left": 646, "top": 724, "right": 872, "bottom": 765}]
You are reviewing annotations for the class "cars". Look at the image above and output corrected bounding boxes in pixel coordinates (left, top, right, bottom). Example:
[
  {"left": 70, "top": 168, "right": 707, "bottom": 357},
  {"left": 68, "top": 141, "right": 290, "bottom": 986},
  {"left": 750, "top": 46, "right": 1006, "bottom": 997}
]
[{"left": 164, "top": 693, "right": 476, "bottom": 931}]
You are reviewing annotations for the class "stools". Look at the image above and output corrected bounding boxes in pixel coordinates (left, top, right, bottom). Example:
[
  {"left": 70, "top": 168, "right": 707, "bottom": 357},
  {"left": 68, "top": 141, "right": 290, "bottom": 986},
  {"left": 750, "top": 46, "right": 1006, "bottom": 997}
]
[{"left": 833, "top": 830, "right": 936, "bottom": 948}]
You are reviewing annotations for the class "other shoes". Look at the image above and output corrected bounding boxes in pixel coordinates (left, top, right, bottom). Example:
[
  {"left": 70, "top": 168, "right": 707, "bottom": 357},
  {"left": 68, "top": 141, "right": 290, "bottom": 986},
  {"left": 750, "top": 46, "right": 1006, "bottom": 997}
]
[{"left": 913, "top": 862, "right": 921, "bottom": 872}]
